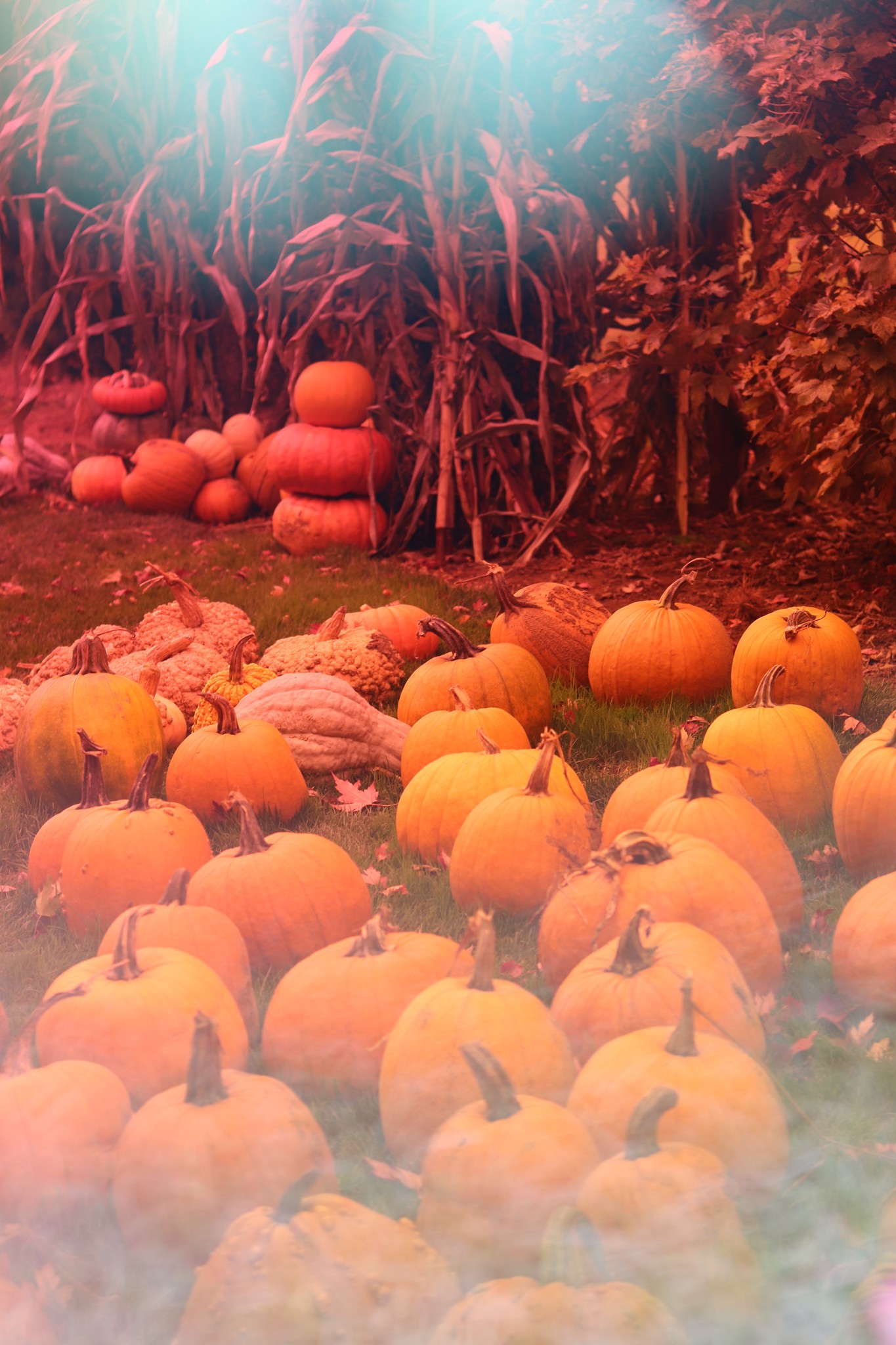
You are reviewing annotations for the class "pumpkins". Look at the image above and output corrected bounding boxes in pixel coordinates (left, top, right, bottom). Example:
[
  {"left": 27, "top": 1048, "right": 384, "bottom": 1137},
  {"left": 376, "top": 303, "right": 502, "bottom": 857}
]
[{"left": 0, "top": 363, "right": 896, "bottom": 1345}]
